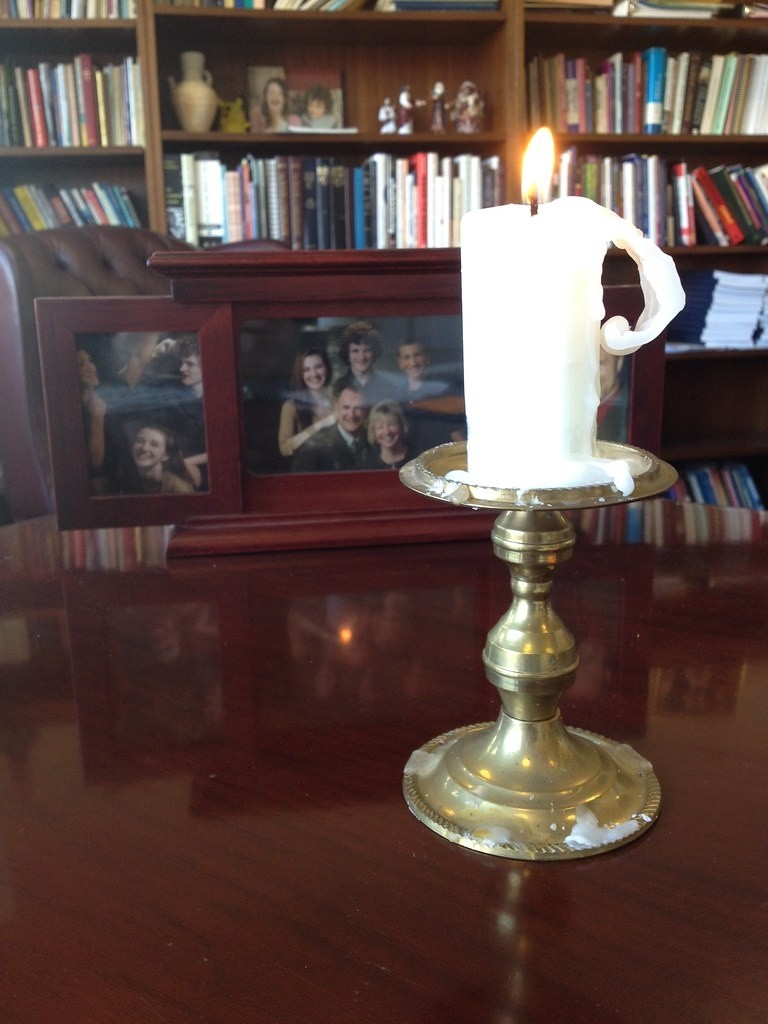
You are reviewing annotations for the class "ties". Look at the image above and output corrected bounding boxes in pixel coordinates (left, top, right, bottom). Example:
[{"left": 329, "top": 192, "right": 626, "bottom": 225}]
[{"left": 352, "top": 440, "right": 360, "bottom": 455}]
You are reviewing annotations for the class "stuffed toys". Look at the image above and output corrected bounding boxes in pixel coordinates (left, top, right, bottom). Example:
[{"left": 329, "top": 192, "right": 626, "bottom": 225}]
[{"left": 216, "top": 93, "right": 251, "bottom": 135}]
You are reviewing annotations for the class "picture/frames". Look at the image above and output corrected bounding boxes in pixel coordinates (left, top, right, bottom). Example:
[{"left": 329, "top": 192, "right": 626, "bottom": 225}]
[
  {"left": 235, "top": 302, "right": 471, "bottom": 513},
  {"left": 32, "top": 295, "right": 243, "bottom": 532}
]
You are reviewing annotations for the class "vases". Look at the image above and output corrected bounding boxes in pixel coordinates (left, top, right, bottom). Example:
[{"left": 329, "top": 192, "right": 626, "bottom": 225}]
[{"left": 169, "top": 50, "right": 218, "bottom": 133}]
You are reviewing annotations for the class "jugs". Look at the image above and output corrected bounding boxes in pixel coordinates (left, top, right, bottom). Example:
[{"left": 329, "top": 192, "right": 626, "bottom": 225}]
[{"left": 167, "top": 51, "right": 217, "bottom": 133}]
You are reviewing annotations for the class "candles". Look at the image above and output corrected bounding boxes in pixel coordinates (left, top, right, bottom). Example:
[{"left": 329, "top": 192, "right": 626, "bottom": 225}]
[{"left": 458, "top": 132, "right": 686, "bottom": 491}]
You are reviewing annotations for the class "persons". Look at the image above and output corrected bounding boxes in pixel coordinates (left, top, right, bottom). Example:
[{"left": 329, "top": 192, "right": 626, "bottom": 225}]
[
  {"left": 277, "top": 319, "right": 465, "bottom": 473},
  {"left": 449, "top": 80, "right": 486, "bottom": 135},
  {"left": 376, "top": 94, "right": 399, "bottom": 135},
  {"left": 428, "top": 80, "right": 454, "bottom": 135},
  {"left": 301, "top": 85, "right": 340, "bottom": 131},
  {"left": 249, "top": 77, "right": 303, "bottom": 132},
  {"left": 74, "top": 328, "right": 212, "bottom": 498},
  {"left": 396, "top": 78, "right": 427, "bottom": 135}
]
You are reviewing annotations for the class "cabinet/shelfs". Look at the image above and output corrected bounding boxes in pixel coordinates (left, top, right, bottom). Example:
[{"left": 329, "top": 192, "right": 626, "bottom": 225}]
[
  {"left": 516, "top": 0, "right": 767, "bottom": 459},
  {"left": 138, "top": 0, "right": 533, "bottom": 253},
  {"left": 0, "top": 0, "right": 152, "bottom": 234}
]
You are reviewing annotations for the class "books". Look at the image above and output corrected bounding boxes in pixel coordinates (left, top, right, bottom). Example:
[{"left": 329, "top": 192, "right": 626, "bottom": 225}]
[
  {"left": 0, "top": 0, "right": 768, "bottom": 253},
  {"left": 665, "top": 458, "right": 768, "bottom": 511},
  {"left": 668, "top": 268, "right": 768, "bottom": 352},
  {"left": 567, "top": 494, "right": 768, "bottom": 551}
]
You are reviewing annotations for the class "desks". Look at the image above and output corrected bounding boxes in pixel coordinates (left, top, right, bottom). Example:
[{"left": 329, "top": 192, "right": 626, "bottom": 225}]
[{"left": 0, "top": 516, "right": 768, "bottom": 1024}]
